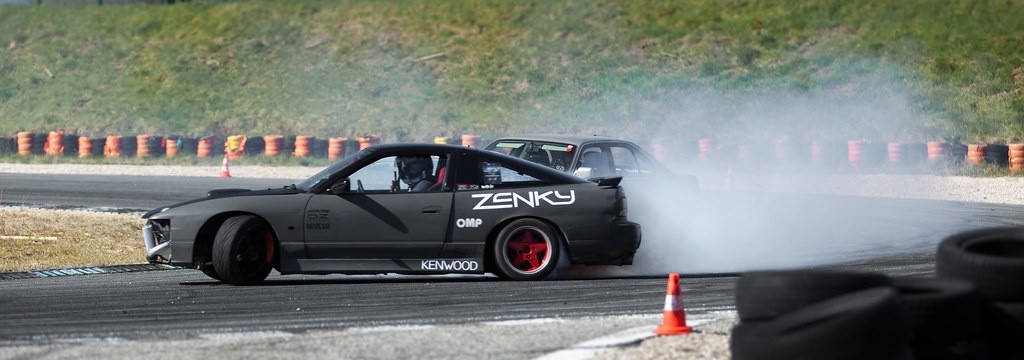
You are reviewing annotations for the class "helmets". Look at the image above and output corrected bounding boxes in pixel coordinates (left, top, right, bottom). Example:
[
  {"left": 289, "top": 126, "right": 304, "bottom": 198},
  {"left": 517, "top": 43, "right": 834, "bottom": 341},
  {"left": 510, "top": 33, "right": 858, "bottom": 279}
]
[
  {"left": 563, "top": 149, "right": 584, "bottom": 169},
  {"left": 394, "top": 153, "right": 433, "bottom": 185}
]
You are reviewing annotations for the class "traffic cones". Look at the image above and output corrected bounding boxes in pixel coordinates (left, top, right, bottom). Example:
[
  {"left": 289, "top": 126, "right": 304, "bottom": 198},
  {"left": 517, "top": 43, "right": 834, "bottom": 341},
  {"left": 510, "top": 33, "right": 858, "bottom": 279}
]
[
  {"left": 653, "top": 273, "right": 693, "bottom": 335},
  {"left": 218, "top": 153, "right": 232, "bottom": 179}
]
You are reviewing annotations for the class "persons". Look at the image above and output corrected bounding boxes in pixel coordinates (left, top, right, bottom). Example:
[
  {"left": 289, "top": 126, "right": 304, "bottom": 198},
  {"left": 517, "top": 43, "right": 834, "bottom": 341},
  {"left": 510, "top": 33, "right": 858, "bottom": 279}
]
[{"left": 391, "top": 156, "right": 435, "bottom": 192}]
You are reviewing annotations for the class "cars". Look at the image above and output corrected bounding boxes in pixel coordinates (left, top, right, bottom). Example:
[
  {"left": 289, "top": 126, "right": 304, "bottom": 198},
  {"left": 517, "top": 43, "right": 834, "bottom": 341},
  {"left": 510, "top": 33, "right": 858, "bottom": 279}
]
[
  {"left": 139, "top": 142, "right": 643, "bottom": 284},
  {"left": 464, "top": 137, "right": 674, "bottom": 222}
]
